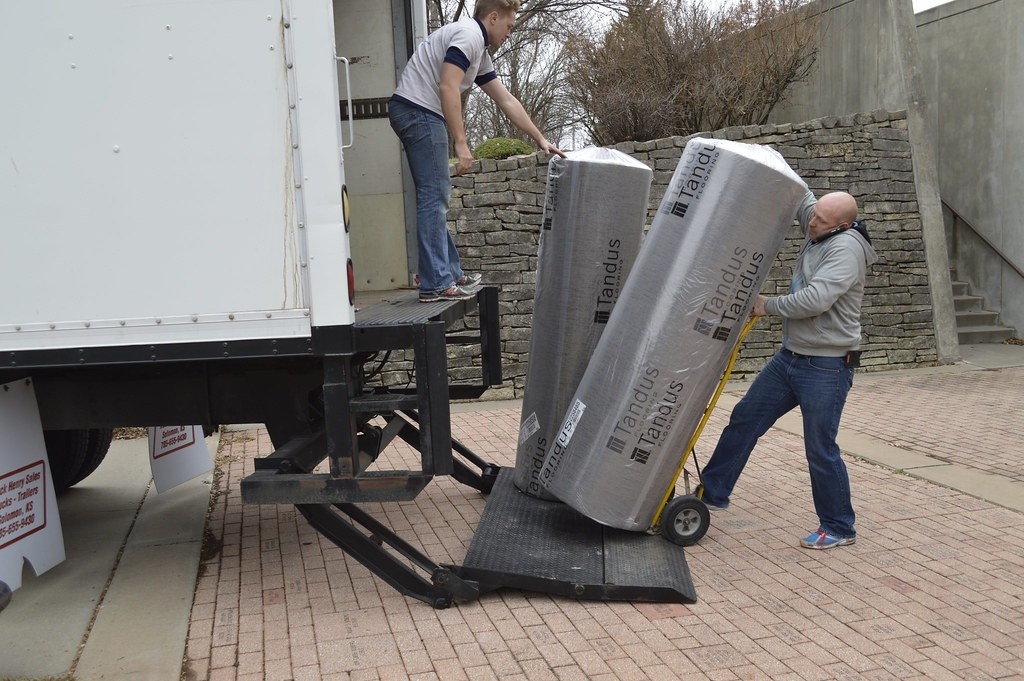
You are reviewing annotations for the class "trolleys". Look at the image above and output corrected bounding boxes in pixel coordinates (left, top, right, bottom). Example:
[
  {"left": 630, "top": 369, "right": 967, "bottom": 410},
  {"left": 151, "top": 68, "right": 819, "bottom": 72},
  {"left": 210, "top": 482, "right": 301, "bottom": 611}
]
[{"left": 644, "top": 306, "right": 765, "bottom": 546}]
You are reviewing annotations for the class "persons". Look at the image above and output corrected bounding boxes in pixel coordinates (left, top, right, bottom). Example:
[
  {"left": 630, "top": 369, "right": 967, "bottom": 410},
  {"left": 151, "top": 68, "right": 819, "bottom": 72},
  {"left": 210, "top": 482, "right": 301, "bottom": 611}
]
[
  {"left": 388, "top": 1, "right": 567, "bottom": 302},
  {"left": 688, "top": 191, "right": 878, "bottom": 549}
]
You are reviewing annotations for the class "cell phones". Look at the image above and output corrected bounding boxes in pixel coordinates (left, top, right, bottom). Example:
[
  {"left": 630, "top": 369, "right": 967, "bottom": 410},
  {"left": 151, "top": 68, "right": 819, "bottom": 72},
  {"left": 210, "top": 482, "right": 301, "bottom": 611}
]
[{"left": 811, "top": 227, "right": 847, "bottom": 244}]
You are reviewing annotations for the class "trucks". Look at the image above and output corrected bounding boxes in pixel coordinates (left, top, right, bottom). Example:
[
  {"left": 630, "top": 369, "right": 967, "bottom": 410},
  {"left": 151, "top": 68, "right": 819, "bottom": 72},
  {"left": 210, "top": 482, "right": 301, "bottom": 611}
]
[{"left": 0, "top": 0, "right": 504, "bottom": 594}]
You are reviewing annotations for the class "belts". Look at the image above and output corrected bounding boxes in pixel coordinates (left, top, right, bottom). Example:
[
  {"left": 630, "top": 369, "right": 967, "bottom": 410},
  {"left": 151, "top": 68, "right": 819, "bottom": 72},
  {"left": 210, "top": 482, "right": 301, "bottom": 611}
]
[{"left": 785, "top": 349, "right": 808, "bottom": 358}]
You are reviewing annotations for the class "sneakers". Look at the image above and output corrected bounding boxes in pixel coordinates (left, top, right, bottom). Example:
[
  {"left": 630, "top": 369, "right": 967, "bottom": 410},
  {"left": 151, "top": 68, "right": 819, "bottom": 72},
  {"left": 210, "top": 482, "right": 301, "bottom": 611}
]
[
  {"left": 418, "top": 283, "right": 476, "bottom": 303},
  {"left": 800, "top": 529, "right": 857, "bottom": 549},
  {"left": 457, "top": 273, "right": 481, "bottom": 288},
  {"left": 690, "top": 484, "right": 729, "bottom": 513}
]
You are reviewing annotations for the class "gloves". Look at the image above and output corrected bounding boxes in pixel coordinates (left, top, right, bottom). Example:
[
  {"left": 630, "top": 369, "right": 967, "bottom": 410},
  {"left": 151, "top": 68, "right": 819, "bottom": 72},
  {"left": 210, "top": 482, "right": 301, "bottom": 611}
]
[{"left": 752, "top": 295, "right": 768, "bottom": 315}]
